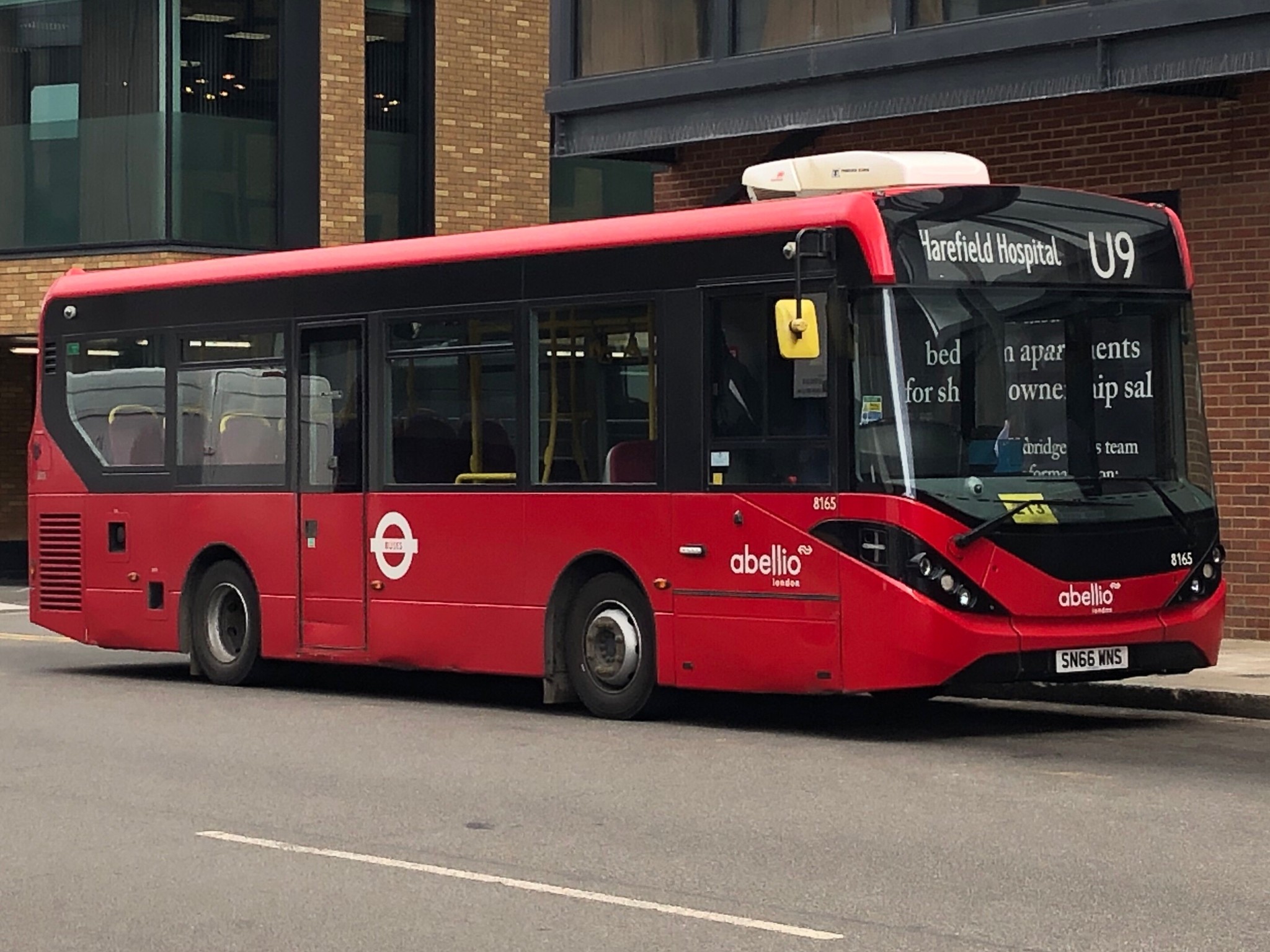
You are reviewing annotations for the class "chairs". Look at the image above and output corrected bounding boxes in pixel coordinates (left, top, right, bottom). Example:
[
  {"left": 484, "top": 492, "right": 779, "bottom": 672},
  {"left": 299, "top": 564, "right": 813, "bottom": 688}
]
[{"left": 107, "top": 403, "right": 659, "bottom": 494}]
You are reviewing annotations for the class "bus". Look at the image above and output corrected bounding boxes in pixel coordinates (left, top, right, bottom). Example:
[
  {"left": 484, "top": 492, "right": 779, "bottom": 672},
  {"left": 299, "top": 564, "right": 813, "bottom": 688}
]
[{"left": 24, "top": 147, "right": 1229, "bottom": 724}]
